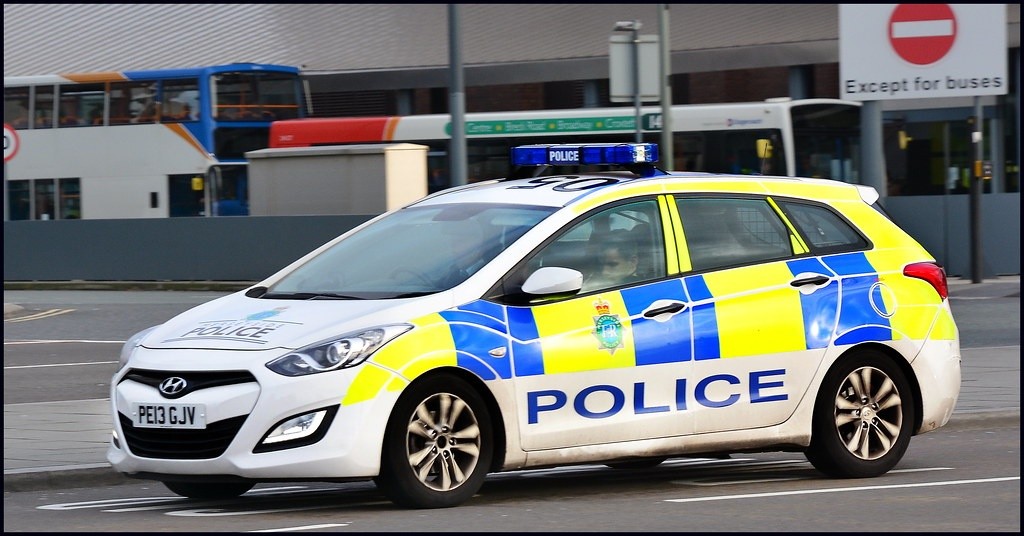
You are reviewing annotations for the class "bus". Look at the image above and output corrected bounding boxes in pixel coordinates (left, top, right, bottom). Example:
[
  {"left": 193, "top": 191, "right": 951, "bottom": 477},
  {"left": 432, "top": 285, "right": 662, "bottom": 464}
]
[
  {"left": 4, "top": 62, "right": 312, "bottom": 221},
  {"left": 264, "top": 95, "right": 926, "bottom": 211}
]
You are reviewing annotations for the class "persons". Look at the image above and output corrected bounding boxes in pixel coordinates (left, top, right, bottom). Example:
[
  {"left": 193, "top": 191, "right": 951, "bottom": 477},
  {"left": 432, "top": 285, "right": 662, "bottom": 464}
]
[
  {"left": 429, "top": 222, "right": 491, "bottom": 288},
  {"left": 183, "top": 102, "right": 192, "bottom": 119},
  {"left": 139, "top": 97, "right": 156, "bottom": 123},
  {"left": 582, "top": 229, "right": 636, "bottom": 291},
  {"left": 18, "top": 101, "right": 119, "bottom": 125},
  {"left": 759, "top": 158, "right": 772, "bottom": 176},
  {"left": 195, "top": 175, "right": 228, "bottom": 205}
]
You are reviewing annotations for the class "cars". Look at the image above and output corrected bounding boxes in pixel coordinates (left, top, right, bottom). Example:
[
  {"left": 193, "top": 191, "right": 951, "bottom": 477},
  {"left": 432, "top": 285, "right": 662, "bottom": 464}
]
[{"left": 104, "top": 140, "right": 961, "bottom": 511}]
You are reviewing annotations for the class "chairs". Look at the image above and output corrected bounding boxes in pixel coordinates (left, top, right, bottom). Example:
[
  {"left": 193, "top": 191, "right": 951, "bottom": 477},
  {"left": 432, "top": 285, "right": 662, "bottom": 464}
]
[{"left": 630, "top": 224, "right": 653, "bottom": 281}]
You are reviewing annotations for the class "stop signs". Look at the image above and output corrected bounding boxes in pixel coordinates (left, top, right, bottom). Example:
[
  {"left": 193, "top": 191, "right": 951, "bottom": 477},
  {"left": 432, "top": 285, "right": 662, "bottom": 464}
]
[{"left": 886, "top": 4, "right": 958, "bottom": 66}]
